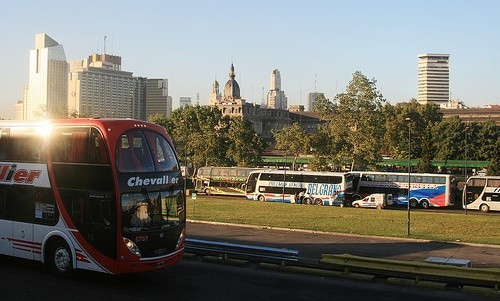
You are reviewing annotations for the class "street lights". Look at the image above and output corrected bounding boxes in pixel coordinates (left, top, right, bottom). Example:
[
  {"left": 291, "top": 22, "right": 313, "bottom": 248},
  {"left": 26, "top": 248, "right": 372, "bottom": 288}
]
[{"left": 404, "top": 118, "right": 414, "bottom": 236}]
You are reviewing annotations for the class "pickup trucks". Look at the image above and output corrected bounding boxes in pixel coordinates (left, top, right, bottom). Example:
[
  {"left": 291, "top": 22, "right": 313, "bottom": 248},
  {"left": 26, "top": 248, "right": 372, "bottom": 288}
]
[{"left": 352, "top": 193, "right": 394, "bottom": 209}]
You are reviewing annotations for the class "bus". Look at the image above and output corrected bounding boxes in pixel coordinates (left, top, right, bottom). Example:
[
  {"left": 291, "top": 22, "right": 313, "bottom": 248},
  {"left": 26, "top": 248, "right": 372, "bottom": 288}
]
[
  {"left": 194, "top": 167, "right": 267, "bottom": 196},
  {"left": 240, "top": 170, "right": 354, "bottom": 208},
  {"left": 0, "top": 117, "right": 188, "bottom": 290},
  {"left": 462, "top": 168, "right": 500, "bottom": 213},
  {"left": 347, "top": 171, "right": 458, "bottom": 210}
]
